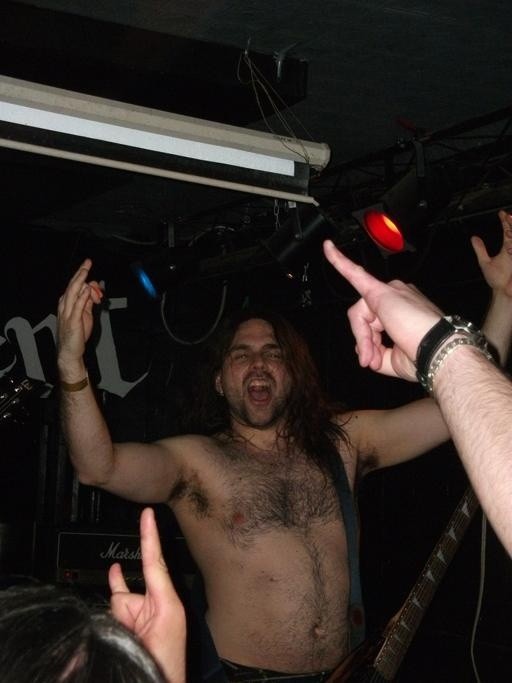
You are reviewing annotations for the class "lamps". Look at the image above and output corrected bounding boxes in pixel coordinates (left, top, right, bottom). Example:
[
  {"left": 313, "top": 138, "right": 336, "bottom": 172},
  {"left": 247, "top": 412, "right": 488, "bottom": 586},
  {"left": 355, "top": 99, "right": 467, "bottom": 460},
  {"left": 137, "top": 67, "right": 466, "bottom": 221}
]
[
  {"left": 257, "top": 207, "right": 336, "bottom": 280},
  {"left": 360, "top": 166, "right": 451, "bottom": 254}
]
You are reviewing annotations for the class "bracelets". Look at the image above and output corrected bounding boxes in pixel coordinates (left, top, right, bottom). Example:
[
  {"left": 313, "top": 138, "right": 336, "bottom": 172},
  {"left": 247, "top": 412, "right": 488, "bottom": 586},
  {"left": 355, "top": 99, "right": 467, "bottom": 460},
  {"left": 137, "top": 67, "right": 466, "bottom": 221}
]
[
  {"left": 60, "top": 367, "right": 89, "bottom": 393},
  {"left": 426, "top": 336, "right": 498, "bottom": 403}
]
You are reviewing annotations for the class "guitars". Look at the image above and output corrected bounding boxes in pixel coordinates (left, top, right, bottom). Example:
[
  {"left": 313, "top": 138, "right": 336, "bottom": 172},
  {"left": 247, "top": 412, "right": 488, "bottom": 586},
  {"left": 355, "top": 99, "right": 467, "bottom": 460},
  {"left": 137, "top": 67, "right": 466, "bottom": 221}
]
[{"left": 328, "top": 486, "right": 479, "bottom": 683}]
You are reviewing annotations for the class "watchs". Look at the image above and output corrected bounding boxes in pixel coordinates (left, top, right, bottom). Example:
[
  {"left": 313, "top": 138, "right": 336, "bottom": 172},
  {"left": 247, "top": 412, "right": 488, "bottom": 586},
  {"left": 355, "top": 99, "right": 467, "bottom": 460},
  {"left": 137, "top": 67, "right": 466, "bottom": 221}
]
[{"left": 415, "top": 312, "right": 487, "bottom": 396}]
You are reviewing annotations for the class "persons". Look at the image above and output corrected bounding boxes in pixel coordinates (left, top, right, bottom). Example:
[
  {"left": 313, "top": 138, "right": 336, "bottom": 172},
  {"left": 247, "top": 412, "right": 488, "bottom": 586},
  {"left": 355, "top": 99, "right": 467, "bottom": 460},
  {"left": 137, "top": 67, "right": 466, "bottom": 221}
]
[
  {"left": 320, "top": 232, "right": 512, "bottom": 562},
  {"left": 1, "top": 506, "right": 192, "bottom": 682},
  {"left": 55, "top": 207, "right": 511, "bottom": 682}
]
